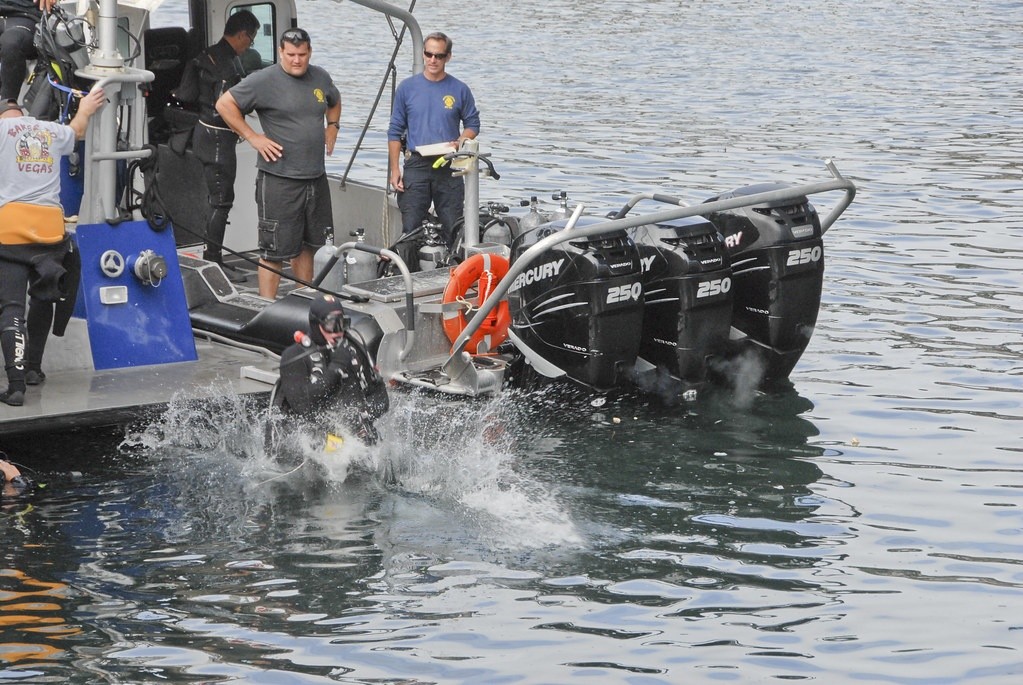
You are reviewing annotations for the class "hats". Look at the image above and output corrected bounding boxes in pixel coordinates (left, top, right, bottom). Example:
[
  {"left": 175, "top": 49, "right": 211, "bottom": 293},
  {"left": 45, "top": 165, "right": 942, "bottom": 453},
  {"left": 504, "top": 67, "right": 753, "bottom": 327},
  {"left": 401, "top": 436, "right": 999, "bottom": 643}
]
[{"left": 0, "top": 98, "right": 21, "bottom": 115}]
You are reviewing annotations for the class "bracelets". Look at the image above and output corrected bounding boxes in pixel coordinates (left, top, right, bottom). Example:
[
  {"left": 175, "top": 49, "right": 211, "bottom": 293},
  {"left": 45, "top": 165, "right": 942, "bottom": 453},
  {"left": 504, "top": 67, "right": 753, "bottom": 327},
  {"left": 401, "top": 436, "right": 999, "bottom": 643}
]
[
  {"left": 328, "top": 122, "right": 340, "bottom": 129},
  {"left": 456, "top": 140, "right": 464, "bottom": 147}
]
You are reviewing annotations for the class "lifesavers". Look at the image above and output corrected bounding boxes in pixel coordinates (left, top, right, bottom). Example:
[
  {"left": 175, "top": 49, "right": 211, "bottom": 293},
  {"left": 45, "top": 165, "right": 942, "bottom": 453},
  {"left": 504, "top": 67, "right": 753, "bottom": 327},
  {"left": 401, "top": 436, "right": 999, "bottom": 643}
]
[{"left": 441, "top": 254, "right": 515, "bottom": 353}]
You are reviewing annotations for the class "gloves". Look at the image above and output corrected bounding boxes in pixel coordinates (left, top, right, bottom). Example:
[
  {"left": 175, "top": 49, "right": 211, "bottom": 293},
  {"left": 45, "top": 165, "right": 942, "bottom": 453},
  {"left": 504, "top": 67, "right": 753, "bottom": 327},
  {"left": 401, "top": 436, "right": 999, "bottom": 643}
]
[{"left": 328, "top": 345, "right": 354, "bottom": 371}]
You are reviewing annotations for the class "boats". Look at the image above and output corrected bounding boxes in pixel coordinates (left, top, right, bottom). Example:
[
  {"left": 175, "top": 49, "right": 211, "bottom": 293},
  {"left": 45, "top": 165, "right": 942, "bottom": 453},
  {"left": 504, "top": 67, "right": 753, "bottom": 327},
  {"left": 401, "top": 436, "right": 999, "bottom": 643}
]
[{"left": 2, "top": 2, "right": 858, "bottom": 440}]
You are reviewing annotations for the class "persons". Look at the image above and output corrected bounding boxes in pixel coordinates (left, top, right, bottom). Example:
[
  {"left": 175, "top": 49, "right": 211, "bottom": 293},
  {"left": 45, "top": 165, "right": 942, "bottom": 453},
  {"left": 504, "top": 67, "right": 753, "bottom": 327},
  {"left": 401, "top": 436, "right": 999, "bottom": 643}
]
[
  {"left": 0, "top": 454, "right": 34, "bottom": 487},
  {"left": 174, "top": 9, "right": 261, "bottom": 282},
  {"left": 216, "top": 28, "right": 341, "bottom": 299},
  {"left": 279, "top": 295, "right": 389, "bottom": 447},
  {"left": 387, "top": 32, "right": 481, "bottom": 242},
  {"left": 0, "top": 87, "right": 106, "bottom": 406},
  {"left": 0, "top": 0, "right": 58, "bottom": 98}
]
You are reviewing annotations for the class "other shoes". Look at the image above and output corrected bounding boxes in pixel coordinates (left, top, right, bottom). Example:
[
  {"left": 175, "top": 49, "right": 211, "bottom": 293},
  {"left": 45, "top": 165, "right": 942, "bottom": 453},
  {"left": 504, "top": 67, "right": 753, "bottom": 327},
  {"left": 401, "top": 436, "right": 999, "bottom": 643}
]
[
  {"left": 204, "top": 251, "right": 247, "bottom": 282},
  {"left": 26, "top": 370, "right": 45, "bottom": 384},
  {"left": 0, "top": 388, "right": 25, "bottom": 406}
]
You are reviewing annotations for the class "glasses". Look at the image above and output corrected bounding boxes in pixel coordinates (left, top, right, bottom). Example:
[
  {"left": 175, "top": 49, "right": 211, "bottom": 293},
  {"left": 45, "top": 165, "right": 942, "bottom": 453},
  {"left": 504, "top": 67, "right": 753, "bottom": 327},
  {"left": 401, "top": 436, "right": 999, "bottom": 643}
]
[
  {"left": 246, "top": 32, "right": 255, "bottom": 46},
  {"left": 316, "top": 314, "right": 344, "bottom": 332},
  {"left": 424, "top": 47, "right": 448, "bottom": 59},
  {"left": 284, "top": 31, "right": 307, "bottom": 41}
]
